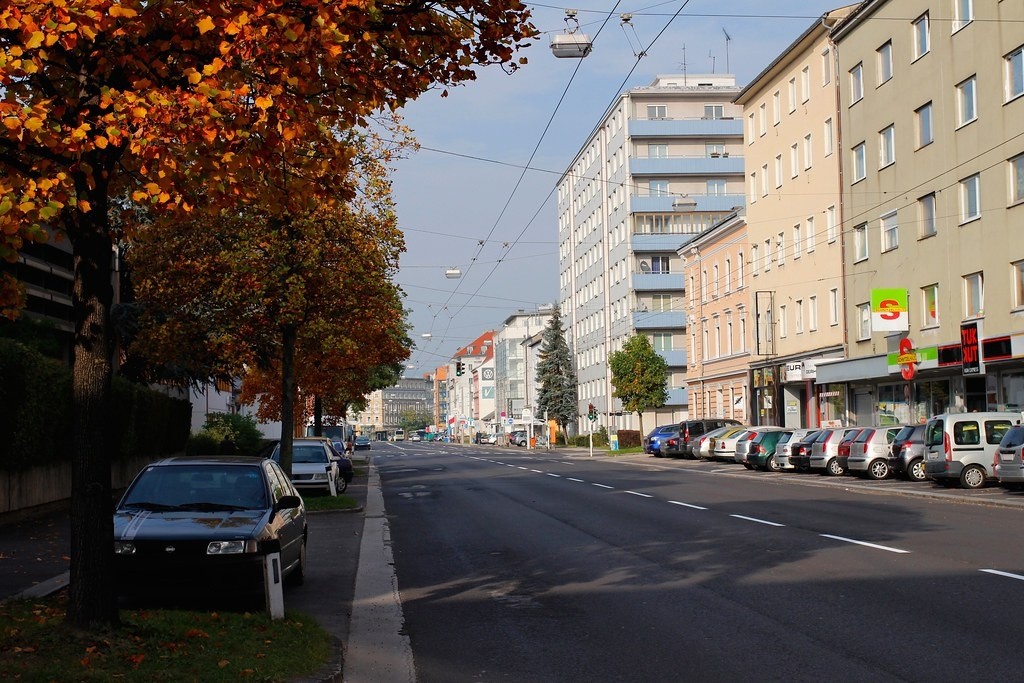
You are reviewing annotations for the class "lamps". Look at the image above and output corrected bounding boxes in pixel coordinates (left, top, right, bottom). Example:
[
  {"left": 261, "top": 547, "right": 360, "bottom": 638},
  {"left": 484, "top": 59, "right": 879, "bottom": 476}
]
[
  {"left": 672, "top": 193, "right": 697, "bottom": 213},
  {"left": 395, "top": 384, "right": 400, "bottom": 388},
  {"left": 400, "top": 375, "right": 406, "bottom": 379},
  {"left": 388, "top": 400, "right": 393, "bottom": 402},
  {"left": 421, "top": 332, "right": 434, "bottom": 342},
  {"left": 407, "top": 365, "right": 416, "bottom": 370},
  {"left": 444, "top": 267, "right": 462, "bottom": 279},
  {"left": 550, "top": 9, "right": 594, "bottom": 58},
  {"left": 391, "top": 393, "right": 396, "bottom": 397}
]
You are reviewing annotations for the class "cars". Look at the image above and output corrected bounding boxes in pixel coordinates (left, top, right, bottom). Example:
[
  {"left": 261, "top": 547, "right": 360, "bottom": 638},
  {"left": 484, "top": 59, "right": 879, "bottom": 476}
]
[
  {"left": 113, "top": 455, "right": 317, "bottom": 605},
  {"left": 268, "top": 437, "right": 348, "bottom": 496},
  {"left": 480, "top": 430, "right": 541, "bottom": 447},
  {"left": 640, "top": 408, "right": 1024, "bottom": 495},
  {"left": 353, "top": 436, "right": 372, "bottom": 451},
  {"left": 412, "top": 435, "right": 421, "bottom": 442}
]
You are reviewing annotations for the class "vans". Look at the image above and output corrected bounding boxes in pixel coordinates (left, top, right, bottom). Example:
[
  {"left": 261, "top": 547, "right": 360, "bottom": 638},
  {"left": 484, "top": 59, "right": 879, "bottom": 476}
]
[{"left": 305, "top": 414, "right": 349, "bottom": 443}]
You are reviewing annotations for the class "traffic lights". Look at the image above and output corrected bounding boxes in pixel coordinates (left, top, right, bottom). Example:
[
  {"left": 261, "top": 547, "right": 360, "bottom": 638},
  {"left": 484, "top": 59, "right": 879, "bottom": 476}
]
[
  {"left": 456, "top": 361, "right": 466, "bottom": 376},
  {"left": 588, "top": 404, "right": 598, "bottom": 421}
]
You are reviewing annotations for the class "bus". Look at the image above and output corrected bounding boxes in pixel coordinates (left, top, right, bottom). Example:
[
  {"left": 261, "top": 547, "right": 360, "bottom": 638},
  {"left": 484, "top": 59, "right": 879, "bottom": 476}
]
[{"left": 395, "top": 430, "right": 405, "bottom": 441}]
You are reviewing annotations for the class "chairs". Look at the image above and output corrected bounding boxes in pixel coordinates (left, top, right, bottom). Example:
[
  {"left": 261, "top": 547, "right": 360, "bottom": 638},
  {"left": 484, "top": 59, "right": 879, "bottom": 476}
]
[
  {"left": 964, "top": 430, "right": 975, "bottom": 442},
  {"left": 334, "top": 443, "right": 341, "bottom": 450},
  {"left": 234, "top": 475, "right": 256, "bottom": 502},
  {"left": 165, "top": 479, "right": 194, "bottom": 503},
  {"left": 311, "top": 451, "right": 325, "bottom": 460}
]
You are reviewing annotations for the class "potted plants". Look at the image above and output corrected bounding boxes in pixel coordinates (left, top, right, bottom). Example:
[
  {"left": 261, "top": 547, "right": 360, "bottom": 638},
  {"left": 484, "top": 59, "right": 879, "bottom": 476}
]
[
  {"left": 723, "top": 151, "right": 730, "bottom": 158},
  {"left": 710, "top": 151, "right": 721, "bottom": 158}
]
